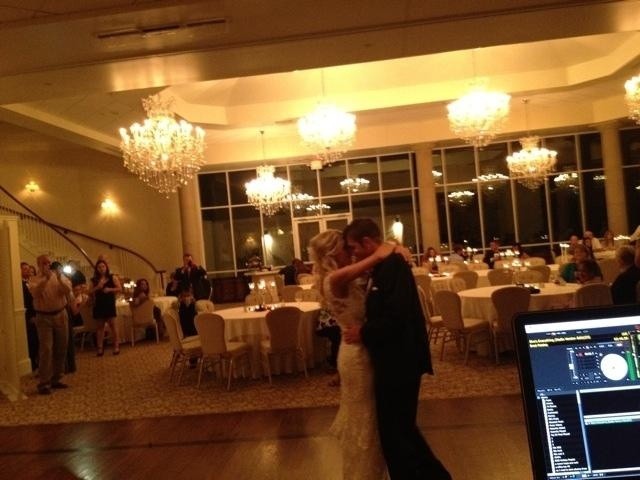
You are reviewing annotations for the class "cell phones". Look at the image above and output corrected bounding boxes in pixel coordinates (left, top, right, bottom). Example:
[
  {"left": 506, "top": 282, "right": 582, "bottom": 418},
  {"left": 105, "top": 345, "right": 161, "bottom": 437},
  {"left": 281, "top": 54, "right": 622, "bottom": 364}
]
[
  {"left": 188, "top": 261, "right": 192, "bottom": 267},
  {"left": 49, "top": 263, "right": 58, "bottom": 270}
]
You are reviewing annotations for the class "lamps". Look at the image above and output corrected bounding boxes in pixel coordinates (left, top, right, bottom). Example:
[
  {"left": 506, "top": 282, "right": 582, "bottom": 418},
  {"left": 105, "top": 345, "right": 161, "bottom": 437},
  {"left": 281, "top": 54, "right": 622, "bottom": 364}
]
[
  {"left": 473, "top": 170, "right": 509, "bottom": 195},
  {"left": 555, "top": 170, "right": 583, "bottom": 193},
  {"left": 447, "top": 190, "right": 475, "bottom": 207},
  {"left": 448, "top": 77, "right": 511, "bottom": 152},
  {"left": 432, "top": 169, "right": 442, "bottom": 185},
  {"left": 503, "top": 112, "right": 561, "bottom": 194},
  {"left": 622, "top": 74, "right": 640, "bottom": 123},
  {"left": 339, "top": 170, "right": 370, "bottom": 193},
  {"left": 244, "top": 141, "right": 292, "bottom": 218},
  {"left": 297, "top": 82, "right": 357, "bottom": 162},
  {"left": 308, "top": 202, "right": 332, "bottom": 217},
  {"left": 281, "top": 187, "right": 313, "bottom": 215},
  {"left": 118, "top": 106, "right": 207, "bottom": 202}
]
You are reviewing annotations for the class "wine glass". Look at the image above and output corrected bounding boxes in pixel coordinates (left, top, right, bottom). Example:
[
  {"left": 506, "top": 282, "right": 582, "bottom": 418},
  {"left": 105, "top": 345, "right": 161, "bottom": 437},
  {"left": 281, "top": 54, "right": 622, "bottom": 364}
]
[{"left": 293, "top": 287, "right": 303, "bottom": 309}]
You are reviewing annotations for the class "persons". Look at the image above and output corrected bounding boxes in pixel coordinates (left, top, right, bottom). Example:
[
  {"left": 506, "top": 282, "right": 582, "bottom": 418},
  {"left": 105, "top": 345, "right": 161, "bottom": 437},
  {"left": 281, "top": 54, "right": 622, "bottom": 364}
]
[
  {"left": 557, "top": 229, "right": 640, "bottom": 305},
  {"left": 21, "top": 253, "right": 208, "bottom": 394},
  {"left": 307, "top": 229, "right": 413, "bottom": 480},
  {"left": 448, "top": 245, "right": 464, "bottom": 264},
  {"left": 512, "top": 245, "right": 529, "bottom": 259},
  {"left": 346, "top": 217, "right": 453, "bottom": 480},
  {"left": 423, "top": 247, "right": 440, "bottom": 270},
  {"left": 280, "top": 258, "right": 300, "bottom": 285},
  {"left": 483, "top": 240, "right": 499, "bottom": 267}
]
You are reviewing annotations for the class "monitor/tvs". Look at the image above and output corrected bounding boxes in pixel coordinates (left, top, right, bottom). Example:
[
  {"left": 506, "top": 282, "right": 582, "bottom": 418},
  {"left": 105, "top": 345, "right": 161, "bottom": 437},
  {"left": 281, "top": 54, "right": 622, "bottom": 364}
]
[{"left": 511, "top": 306, "right": 640, "bottom": 480}]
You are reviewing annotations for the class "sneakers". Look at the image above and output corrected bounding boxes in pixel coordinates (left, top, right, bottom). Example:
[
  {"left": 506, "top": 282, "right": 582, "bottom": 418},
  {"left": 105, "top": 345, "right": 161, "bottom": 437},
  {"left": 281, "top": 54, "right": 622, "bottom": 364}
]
[{"left": 39, "top": 382, "right": 68, "bottom": 394}]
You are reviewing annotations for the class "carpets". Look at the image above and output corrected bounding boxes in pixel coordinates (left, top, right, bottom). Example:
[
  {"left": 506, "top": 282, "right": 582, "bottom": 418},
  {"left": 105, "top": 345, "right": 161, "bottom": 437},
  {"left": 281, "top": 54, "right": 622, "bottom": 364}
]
[{"left": 0, "top": 301, "right": 522, "bottom": 429}]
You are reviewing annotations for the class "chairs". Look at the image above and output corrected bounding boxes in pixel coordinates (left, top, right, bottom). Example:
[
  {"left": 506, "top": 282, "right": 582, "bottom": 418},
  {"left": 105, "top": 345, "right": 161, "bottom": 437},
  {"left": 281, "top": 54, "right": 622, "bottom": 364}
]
[
  {"left": 192, "top": 314, "right": 255, "bottom": 387},
  {"left": 79, "top": 288, "right": 178, "bottom": 349},
  {"left": 193, "top": 232, "right": 640, "bottom": 319},
  {"left": 433, "top": 292, "right": 494, "bottom": 367},
  {"left": 416, "top": 285, "right": 448, "bottom": 350},
  {"left": 489, "top": 286, "right": 531, "bottom": 368},
  {"left": 160, "top": 308, "right": 204, "bottom": 385},
  {"left": 257, "top": 307, "right": 311, "bottom": 386}
]
